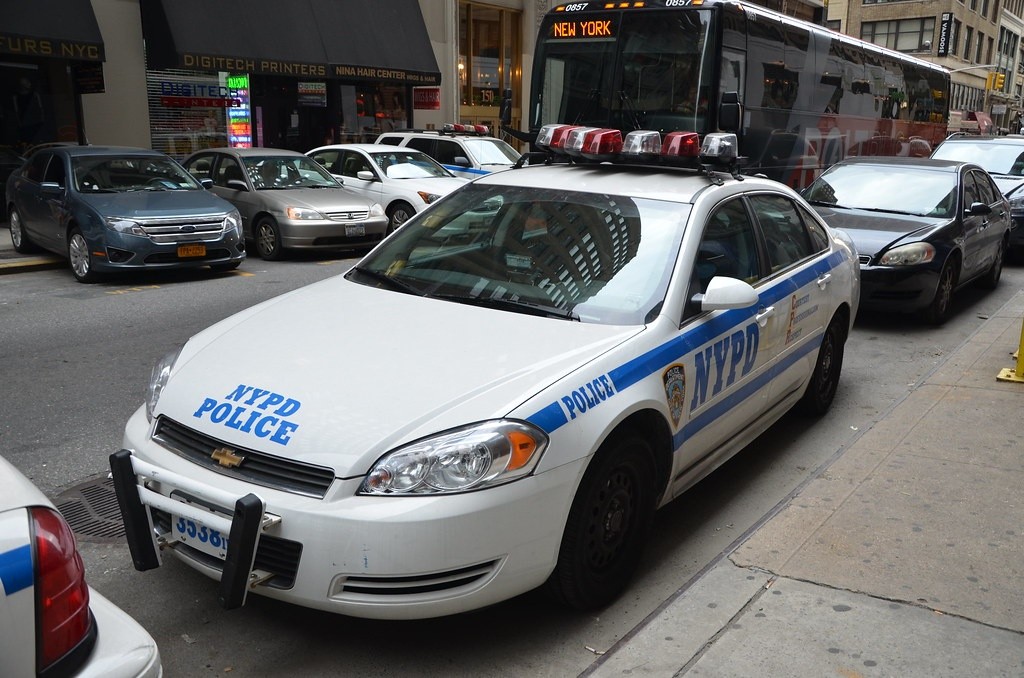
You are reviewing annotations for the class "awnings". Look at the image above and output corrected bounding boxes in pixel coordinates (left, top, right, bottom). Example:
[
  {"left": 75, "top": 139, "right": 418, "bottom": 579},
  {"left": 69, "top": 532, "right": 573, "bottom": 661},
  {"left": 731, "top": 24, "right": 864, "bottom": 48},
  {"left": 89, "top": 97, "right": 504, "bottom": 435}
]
[
  {"left": 0, "top": 0, "right": 107, "bottom": 63},
  {"left": 140, "top": 0, "right": 441, "bottom": 87}
]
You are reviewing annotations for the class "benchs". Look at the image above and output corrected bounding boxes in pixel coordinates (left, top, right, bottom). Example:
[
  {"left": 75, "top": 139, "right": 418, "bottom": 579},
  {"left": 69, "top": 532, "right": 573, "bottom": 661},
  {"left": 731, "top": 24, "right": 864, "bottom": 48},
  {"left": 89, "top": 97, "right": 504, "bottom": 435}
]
[{"left": 194, "top": 161, "right": 226, "bottom": 187}]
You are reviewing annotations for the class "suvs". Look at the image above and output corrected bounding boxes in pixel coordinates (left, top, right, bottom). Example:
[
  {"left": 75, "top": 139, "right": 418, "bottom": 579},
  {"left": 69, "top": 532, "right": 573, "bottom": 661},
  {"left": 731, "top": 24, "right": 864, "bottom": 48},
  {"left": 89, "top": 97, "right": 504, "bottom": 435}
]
[
  {"left": 928, "top": 132, "right": 1024, "bottom": 267},
  {"left": 372, "top": 129, "right": 529, "bottom": 181},
  {"left": 847, "top": 136, "right": 933, "bottom": 158}
]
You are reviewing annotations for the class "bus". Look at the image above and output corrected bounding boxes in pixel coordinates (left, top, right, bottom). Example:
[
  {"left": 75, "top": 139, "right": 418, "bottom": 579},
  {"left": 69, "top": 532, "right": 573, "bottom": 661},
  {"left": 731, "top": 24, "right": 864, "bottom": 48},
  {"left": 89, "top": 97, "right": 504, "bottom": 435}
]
[{"left": 500, "top": 0, "right": 951, "bottom": 196}]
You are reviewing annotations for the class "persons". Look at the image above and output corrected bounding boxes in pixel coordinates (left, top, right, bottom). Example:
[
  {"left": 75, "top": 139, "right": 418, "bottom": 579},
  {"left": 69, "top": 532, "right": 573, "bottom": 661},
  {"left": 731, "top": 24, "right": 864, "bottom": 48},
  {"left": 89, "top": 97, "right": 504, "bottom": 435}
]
[{"left": 674, "top": 85, "right": 709, "bottom": 113}]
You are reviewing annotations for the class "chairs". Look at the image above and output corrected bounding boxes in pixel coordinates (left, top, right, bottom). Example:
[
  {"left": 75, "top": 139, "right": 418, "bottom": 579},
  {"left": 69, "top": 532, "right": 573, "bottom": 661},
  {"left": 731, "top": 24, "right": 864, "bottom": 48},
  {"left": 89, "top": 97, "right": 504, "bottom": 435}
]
[{"left": 312, "top": 156, "right": 326, "bottom": 171}]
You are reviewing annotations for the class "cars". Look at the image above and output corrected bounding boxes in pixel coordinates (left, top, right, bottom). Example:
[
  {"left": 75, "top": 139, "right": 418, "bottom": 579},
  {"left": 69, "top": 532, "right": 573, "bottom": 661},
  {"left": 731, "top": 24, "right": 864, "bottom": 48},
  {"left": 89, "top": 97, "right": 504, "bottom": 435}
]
[
  {"left": 6, "top": 146, "right": 247, "bottom": 283},
  {"left": 256, "top": 143, "right": 503, "bottom": 244},
  {"left": 178, "top": 147, "right": 389, "bottom": 261},
  {"left": 110, "top": 161, "right": 859, "bottom": 619},
  {"left": 0, "top": 456, "right": 163, "bottom": 678},
  {"left": 767, "top": 156, "right": 1012, "bottom": 324},
  {"left": 742, "top": 127, "right": 819, "bottom": 190}
]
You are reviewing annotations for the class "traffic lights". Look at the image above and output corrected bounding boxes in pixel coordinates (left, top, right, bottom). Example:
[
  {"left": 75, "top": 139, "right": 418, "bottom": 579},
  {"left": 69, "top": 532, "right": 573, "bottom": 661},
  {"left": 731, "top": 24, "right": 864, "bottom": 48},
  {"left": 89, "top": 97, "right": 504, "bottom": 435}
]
[
  {"left": 994, "top": 72, "right": 1005, "bottom": 89},
  {"left": 937, "top": 91, "right": 942, "bottom": 98}
]
